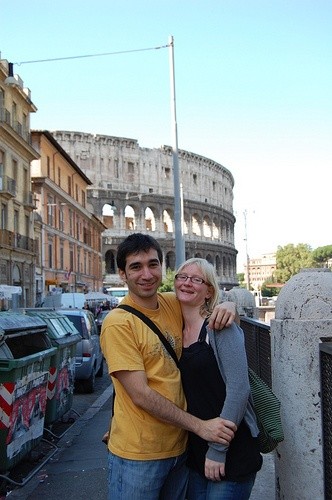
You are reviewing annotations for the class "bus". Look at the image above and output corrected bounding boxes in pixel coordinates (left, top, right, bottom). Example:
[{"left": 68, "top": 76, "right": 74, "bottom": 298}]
[
  {"left": 84, "top": 291, "right": 118, "bottom": 318},
  {"left": 105, "top": 286, "right": 128, "bottom": 306}
]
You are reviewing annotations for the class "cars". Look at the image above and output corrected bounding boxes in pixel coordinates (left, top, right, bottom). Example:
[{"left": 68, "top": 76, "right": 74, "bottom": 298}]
[{"left": 95, "top": 310, "right": 111, "bottom": 329}]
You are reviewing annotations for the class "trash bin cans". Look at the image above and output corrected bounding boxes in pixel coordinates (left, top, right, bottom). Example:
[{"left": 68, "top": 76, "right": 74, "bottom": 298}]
[
  {"left": 0, "top": 314, "right": 57, "bottom": 473},
  {"left": 30, "top": 312, "right": 82, "bottom": 431}
]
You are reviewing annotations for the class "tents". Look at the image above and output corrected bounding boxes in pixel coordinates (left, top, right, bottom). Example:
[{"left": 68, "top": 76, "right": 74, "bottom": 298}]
[{"left": 83, "top": 292, "right": 116, "bottom": 311}]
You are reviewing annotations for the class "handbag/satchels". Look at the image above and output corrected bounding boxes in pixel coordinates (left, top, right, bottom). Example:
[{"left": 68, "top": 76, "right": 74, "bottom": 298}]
[{"left": 248, "top": 365, "right": 283, "bottom": 453}]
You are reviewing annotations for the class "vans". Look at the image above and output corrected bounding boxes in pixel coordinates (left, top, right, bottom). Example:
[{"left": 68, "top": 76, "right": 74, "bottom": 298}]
[
  {"left": 40, "top": 292, "right": 86, "bottom": 311},
  {"left": 56, "top": 309, "right": 103, "bottom": 392}
]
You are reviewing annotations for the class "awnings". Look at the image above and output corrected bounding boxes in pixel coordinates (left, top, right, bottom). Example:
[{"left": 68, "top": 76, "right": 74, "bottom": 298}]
[{"left": 0, "top": 284, "right": 22, "bottom": 295}]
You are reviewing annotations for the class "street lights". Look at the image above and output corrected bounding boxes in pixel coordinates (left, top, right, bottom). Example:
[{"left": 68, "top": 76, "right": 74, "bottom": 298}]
[{"left": 235, "top": 208, "right": 256, "bottom": 291}]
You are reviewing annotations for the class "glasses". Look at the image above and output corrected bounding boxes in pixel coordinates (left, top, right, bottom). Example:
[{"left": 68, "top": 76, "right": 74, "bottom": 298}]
[{"left": 175, "top": 273, "right": 212, "bottom": 288}]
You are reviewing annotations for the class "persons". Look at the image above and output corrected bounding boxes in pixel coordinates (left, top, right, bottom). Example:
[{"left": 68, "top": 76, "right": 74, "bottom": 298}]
[
  {"left": 172, "top": 255, "right": 263, "bottom": 500},
  {"left": 98, "top": 233, "right": 239, "bottom": 500}
]
